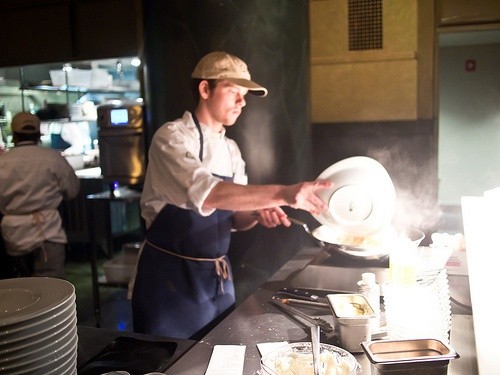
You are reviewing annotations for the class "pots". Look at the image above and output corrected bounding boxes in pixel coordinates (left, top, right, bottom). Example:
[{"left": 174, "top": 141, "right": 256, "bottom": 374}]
[{"left": 253, "top": 213, "right": 424, "bottom": 260}]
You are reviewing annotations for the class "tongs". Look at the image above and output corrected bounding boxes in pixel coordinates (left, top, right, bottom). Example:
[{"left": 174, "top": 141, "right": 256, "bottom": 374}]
[
  {"left": 279, "top": 288, "right": 355, "bottom": 301},
  {"left": 271, "top": 293, "right": 333, "bottom": 342}
]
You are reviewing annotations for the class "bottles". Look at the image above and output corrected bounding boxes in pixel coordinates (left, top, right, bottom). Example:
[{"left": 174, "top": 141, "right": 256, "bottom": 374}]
[{"left": 357, "top": 274, "right": 380, "bottom": 332}]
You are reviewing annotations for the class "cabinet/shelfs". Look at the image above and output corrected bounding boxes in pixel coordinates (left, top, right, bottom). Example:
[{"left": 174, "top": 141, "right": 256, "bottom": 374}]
[{"left": 86, "top": 186, "right": 146, "bottom": 327}]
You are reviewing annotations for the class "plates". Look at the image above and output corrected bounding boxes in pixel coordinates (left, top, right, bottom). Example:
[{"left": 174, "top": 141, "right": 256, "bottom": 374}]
[
  {"left": 0, "top": 277, "right": 77, "bottom": 375},
  {"left": 309, "top": 155, "right": 395, "bottom": 236},
  {"left": 384, "top": 270, "right": 452, "bottom": 343}
]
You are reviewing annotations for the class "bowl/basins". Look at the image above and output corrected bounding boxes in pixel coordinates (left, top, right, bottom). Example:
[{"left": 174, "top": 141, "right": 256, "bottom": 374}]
[{"left": 260, "top": 341, "right": 360, "bottom": 375}]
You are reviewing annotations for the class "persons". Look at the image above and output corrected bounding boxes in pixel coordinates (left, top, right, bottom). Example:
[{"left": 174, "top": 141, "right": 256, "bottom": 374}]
[
  {"left": 0, "top": 112, "right": 81, "bottom": 277},
  {"left": 126, "top": 51, "right": 334, "bottom": 338}
]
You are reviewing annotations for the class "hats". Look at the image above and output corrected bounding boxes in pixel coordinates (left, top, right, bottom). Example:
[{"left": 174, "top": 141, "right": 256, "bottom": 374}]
[
  {"left": 192, "top": 51, "right": 268, "bottom": 97},
  {"left": 11, "top": 112, "right": 40, "bottom": 134}
]
